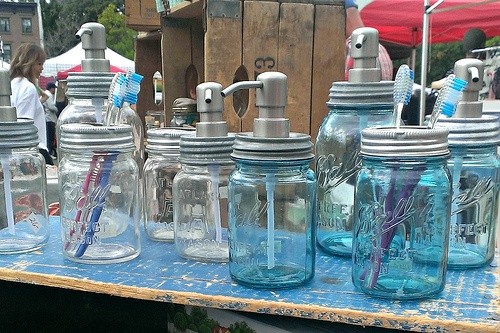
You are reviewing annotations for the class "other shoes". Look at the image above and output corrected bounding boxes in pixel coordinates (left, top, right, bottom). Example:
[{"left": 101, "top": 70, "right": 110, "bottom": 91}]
[{"left": 49, "top": 154, "right": 57, "bottom": 159}]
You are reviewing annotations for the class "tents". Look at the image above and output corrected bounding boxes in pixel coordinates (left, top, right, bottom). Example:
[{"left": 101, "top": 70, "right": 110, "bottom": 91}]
[
  {"left": 355, "top": 0, "right": 500, "bottom": 125},
  {"left": 38, "top": 39, "right": 135, "bottom": 88}
]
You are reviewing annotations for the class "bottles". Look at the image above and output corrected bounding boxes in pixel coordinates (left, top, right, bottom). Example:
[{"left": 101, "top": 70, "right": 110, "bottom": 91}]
[
  {"left": 351, "top": 126, "right": 452, "bottom": 299},
  {"left": 142, "top": 126, "right": 215, "bottom": 243},
  {"left": 57, "top": 124, "right": 140, "bottom": 265}
]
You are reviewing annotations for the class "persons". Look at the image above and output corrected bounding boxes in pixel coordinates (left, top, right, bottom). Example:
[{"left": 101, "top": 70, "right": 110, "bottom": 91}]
[
  {"left": 9, "top": 43, "right": 54, "bottom": 166},
  {"left": 38, "top": 86, "right": 49, "bottom": 104},
  {"left": 345, "top": 0, "right": 393, "bottom": 84},
  {"left": 39, "top": 82, "right": 59, "bottom": 160}
]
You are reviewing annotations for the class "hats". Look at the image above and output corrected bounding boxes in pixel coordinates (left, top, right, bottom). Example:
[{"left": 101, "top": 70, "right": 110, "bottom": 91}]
[{"left": 46, "top": 82, "right": 58, "bottom": 88}]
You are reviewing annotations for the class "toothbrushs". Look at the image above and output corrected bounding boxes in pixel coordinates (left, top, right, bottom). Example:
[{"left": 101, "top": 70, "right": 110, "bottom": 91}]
[
  {"left": 63, "top": 71, "right": 129, "bottom": 252},
  {"left": 368, "top": 64, "right": 415, "bottom": 289},
  {"left": 73, "top": 70, "right": 144, "bottom": 258},
  {"left": 359, "top": 74, "right": 468, "bottom": 281}
]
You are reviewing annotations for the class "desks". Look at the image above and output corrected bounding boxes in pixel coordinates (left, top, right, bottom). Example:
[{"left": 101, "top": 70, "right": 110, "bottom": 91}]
[{"left": 0, "top": 213, "right": 500, "bottom": 333}]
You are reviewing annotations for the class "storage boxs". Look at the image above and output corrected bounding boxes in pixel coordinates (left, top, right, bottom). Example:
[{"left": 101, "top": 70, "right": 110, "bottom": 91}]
[{"left": 125, "top": 0, "right": 347, "bottom": 176}]
